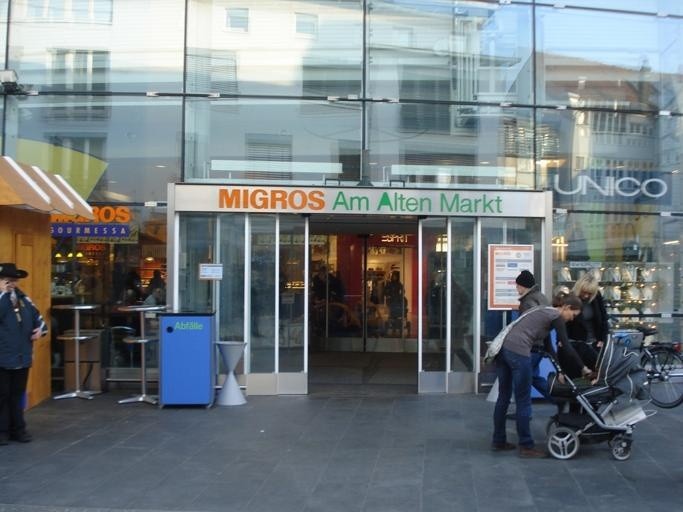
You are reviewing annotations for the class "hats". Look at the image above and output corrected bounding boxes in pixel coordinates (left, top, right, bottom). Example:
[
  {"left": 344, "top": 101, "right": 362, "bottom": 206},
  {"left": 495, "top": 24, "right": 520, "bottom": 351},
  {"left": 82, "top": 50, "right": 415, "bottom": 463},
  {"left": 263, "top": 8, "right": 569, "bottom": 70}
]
[
  {"left": 0, "top": 261, "right": 28, "bottom": 278},
  {"left": 515, "top": 269, "right": 536, "bottom": 288}
]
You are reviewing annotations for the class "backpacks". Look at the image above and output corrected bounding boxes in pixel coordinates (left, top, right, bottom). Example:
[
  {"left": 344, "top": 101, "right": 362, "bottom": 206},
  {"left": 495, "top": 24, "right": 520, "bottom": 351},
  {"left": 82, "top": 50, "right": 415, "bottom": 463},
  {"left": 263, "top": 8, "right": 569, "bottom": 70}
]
[{"left": 482, "top": 305, "right": 563, "bottom": 362}]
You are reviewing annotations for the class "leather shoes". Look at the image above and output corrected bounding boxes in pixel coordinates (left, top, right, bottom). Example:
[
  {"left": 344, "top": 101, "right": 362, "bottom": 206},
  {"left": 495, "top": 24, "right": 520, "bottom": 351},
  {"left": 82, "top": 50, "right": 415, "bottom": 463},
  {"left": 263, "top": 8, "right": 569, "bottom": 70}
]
[
  {"left": 11, "top": 432, "right": 33, "bottom": 443},
  {"left": 490, "top": 441, "right": 516, "bottom": 453},
  {"left": 519, "top": 447, "right": 548, "bottom": 458},
  {"left": 506, "top": 411, "right": 533, "bottom": 422},
  {"left": 0, "top": 435, "right": 10, "bottom": 447}
]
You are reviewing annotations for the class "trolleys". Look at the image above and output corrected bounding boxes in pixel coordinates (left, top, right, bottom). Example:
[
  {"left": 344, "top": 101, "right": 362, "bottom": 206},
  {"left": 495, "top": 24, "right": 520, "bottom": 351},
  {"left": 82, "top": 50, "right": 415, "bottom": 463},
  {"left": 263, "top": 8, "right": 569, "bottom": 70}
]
[{"left": 386, "top": 294, "right": 412, "bottom": 337}]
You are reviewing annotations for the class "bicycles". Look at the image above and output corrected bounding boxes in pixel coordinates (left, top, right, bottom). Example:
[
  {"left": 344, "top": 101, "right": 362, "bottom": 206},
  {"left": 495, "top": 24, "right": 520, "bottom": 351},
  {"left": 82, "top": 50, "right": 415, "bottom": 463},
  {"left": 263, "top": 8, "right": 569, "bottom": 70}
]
[{"left": 607, "top": 314, "right": 683, "bottom": 408}]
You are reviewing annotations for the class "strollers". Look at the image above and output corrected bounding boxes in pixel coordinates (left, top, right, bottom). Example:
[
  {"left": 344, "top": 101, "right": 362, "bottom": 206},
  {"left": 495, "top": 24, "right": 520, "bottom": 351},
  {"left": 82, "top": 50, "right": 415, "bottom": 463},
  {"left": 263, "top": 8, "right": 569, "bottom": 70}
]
[{"left": 544, "top": 330, "right": 658, "bottom": 461}]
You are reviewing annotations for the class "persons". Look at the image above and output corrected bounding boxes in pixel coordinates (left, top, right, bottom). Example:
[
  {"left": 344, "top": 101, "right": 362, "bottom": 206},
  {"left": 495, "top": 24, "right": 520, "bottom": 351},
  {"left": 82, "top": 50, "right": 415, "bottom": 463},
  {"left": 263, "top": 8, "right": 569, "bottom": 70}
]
[
  {"left": 148, "top": 268, "right": 166, "bottom": 292},
  {"left": 142, "top": 288, "right": 163, "bottom": 307},
  {"left": 556, "top": 271, "right": 612, "bottom": 385},
  {"left": 386, "top": 269, "right": 406, "bottom": 322},
  {"left": 505, "top": 269, "right": 568, "bottom": 420},
  {"left": 111, "top": 273, "right": 140, "bottom": 358},
  {"left": 443, "top": 276, "right": 473, "bottom": 371},
  {"left": 491, "top": 291, "right": 592, "bottom": 457},
  {"left": 0, "top": 263, "right": 48, "bottom": 446},
  {"left": 315, "top": 267, "right": 336, "bottom": 299}
]
